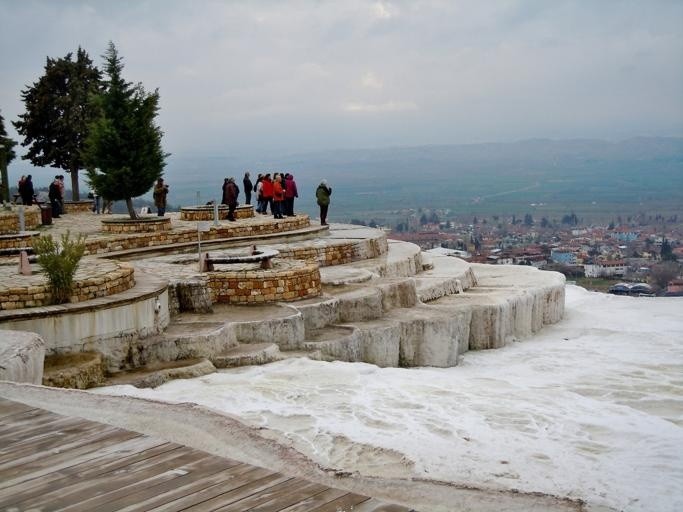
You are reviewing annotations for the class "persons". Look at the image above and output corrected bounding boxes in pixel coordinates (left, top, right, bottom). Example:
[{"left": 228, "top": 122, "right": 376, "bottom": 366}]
[
  {"left": 87, "top": 185, "right": 113, "bottom": 215},
  {"left": 315, "top": 178, "right": 332, "bottom": 226},
  {"left": 17, "top": 175, "right": 35, "bottom": 207},
  {"left": 57, "top": 175, "right": 67, "bottom": 215},
  {"left": 47, "top": 175, "right": 62, "bottom": 218},
  {"left": 219, "top": 172, "right": 299, "bottom": 222},
  {"left": 153, "top": 177, "right": 169, "bottom": 217}
]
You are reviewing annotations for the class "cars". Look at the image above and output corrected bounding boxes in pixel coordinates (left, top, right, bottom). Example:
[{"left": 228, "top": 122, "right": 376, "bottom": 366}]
[{"left": 607, "top": 281, "right": 654, "bottom": 295}]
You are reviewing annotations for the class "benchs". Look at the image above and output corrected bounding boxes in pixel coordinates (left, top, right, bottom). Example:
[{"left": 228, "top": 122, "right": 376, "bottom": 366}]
[{"left": 199, "top": 244, "right": 280, "bottom": 272}]
